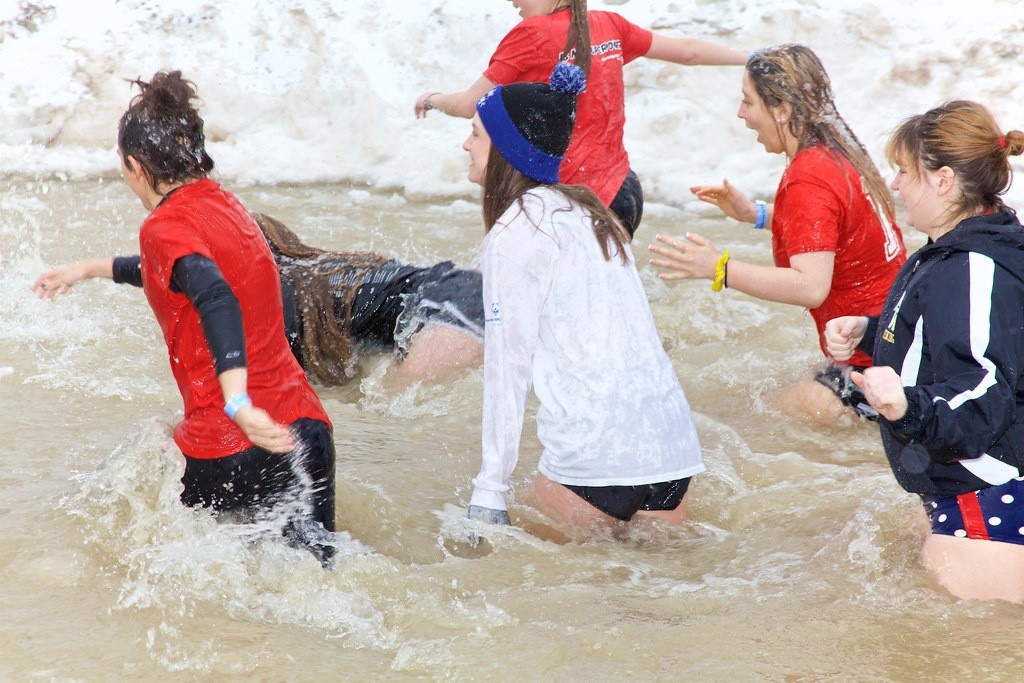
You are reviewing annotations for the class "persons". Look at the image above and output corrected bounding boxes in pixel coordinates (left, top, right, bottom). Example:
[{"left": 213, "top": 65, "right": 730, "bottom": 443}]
[
  {"left": 823, "top": 98, "right": 1024, "bottom": 612},
  {"left": 414, "top": 0, "right": 753, "bottom": 243},
  {"left": 27, "top": 66, "right": 338, "bottom": 579},
  {"left": 645, "top": 41, "right": 908, "bottom": 434},
  {"left": 462, "top": 58, "right": 708, "bottom": 550},
  {"left": 247, "top": 211, "right": 484, "bottom": 405}
]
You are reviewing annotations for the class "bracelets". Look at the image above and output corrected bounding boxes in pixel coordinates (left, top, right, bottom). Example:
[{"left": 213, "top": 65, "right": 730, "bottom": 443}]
[
  {"left": 746, "top": 50, "right": 753, "bottom": 61},
  {"left": 710, "top": 248, "right": 732, "bottom": 292},
  {"left": 223, "top": 391, "right": 253, "bottom": 422},
  {"left": 752, "top": 199, "right": 769, "bottom": 229},
  {"left": 724, "top": 258, "right": 730, "bottom": 289}
]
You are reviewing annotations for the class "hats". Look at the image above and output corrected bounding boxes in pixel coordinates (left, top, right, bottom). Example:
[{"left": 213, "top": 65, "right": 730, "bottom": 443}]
[{"left": 477, "top": 62, "right": 586, "bottom": 185}]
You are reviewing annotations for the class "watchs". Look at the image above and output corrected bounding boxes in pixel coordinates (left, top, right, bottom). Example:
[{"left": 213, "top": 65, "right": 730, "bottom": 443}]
[{"left": 422, "top": 93, "right": 441, "bottom": 111}]
